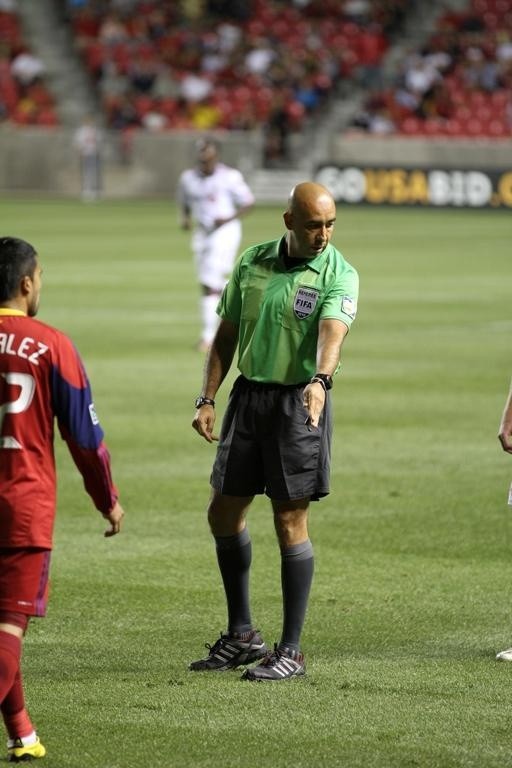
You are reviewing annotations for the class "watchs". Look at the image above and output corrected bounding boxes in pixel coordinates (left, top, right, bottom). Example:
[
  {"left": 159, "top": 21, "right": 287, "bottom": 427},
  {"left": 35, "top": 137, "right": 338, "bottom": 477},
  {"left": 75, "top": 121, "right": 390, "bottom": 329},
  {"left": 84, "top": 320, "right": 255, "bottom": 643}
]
[
  {"left": 195, "top": 397, "right": 214, "bottom": 409},
  {"left": 311, "top": 374, "right": 333, "bottom": 392}
]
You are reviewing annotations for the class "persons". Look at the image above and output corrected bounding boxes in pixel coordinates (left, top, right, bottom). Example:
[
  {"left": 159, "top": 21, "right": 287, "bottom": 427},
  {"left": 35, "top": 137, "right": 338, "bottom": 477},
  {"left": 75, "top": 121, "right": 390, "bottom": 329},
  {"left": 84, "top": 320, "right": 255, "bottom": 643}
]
[
  {"left": 0, "top": 237, "right": 125, "bottom": 762},
  {"left": 2, "top": 0, "right": 511, "bottom": 139},
  {"left": 496, "top": 379, "right": 512, "bottom": 662},
  {"left": 72, "top": 113, "right": 141, "bottom": 203},
  {"left": 189, "top": 183, "right": 359, "bottom": 681},
  {"left": 176, "top": 135, "right": 255, "bottom": 351}
]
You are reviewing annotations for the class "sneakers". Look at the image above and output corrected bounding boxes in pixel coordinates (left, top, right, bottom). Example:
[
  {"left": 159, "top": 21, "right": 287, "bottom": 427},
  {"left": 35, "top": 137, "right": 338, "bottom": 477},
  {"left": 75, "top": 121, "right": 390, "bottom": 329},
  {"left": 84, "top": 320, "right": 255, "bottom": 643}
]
[
  {"left": 495, "top": 648, "right": 512, "bottom": 661},
  {"left": 188, "top": 629, "right": 306, "bottom": 682},
  {"left": 7, "top": 735, "right": 46, "bottom": 762}
]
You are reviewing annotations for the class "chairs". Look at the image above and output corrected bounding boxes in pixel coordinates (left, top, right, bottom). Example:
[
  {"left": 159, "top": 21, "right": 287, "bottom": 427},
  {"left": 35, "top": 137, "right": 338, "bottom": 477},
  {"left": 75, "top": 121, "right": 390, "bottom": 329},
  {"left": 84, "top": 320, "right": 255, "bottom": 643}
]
[{"left": 0, "top": 0, "right": 512, "bottom": 136}]
